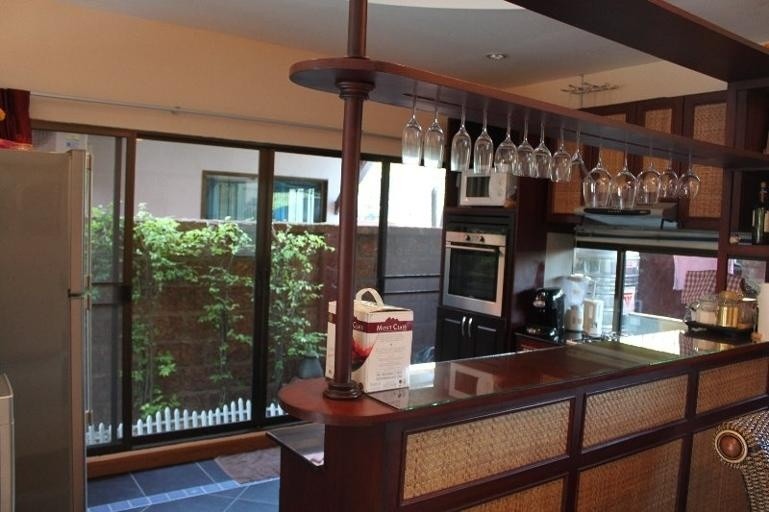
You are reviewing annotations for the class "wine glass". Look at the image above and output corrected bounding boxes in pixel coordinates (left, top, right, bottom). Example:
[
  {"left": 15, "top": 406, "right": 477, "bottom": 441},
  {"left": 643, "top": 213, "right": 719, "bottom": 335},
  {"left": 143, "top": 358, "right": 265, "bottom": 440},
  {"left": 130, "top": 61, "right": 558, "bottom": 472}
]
[
  {"left": 583, "top": 138, "right": 703, "bottom": 210},
  {"left": 402, "top": 98, "right": 586, "bottom": 182}
]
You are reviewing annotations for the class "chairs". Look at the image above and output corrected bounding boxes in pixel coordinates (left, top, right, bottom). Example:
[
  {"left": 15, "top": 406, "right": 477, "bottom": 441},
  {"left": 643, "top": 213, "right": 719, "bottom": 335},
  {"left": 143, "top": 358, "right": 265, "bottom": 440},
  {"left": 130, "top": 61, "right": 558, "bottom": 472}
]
[{"left": 712, "top": 406, "right": 769, "bottom": 511}]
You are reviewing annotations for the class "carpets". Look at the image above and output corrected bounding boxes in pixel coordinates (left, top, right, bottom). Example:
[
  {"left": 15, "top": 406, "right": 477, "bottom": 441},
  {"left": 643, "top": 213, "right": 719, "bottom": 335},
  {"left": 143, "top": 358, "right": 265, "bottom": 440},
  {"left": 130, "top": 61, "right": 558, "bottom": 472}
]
[{"left": 214, "top": 445, "right": 282, "bottom": 484}]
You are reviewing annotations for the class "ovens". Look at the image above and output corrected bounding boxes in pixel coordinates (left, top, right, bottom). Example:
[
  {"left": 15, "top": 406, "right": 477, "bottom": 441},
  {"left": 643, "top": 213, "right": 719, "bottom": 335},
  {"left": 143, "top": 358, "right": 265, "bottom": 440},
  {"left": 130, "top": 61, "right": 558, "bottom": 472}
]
[{"left": 440, "top": 230, "right": 508, "bottom": 320}]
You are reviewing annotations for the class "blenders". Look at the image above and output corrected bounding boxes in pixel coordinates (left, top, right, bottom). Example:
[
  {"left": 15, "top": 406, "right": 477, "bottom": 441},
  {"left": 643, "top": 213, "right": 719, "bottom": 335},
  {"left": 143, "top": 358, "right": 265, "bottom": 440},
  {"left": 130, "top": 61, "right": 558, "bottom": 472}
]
[{"left": 565, "top": 272, "right": 590, "bottom": 332}]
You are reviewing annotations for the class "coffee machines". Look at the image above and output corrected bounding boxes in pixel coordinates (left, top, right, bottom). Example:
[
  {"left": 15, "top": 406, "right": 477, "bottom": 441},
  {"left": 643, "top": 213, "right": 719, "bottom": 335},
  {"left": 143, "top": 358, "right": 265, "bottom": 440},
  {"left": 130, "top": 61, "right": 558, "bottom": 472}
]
[{"left": 526, "top": 287, "right": 565, "bottom": 337}]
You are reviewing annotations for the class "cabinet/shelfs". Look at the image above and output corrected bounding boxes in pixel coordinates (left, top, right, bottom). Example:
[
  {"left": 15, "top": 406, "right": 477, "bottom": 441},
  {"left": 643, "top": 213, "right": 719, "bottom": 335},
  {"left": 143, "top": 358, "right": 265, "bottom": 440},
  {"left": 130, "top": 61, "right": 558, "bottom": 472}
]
[
  {"left": 444, "top": 116, "right": 549, "bottom": 212},
  {"left": 729, "top": 76, "right": 769, "bottom": 257},
  {"left": 435, "top": 306, "right": 506, "bottom": 361}
]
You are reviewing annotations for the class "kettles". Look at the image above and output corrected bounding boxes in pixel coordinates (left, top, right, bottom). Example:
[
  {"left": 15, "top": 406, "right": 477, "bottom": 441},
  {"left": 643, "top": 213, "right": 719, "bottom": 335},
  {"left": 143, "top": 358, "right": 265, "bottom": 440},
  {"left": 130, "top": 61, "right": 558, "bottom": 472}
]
[{"left": 584, "top": 300, "right": 604, "bottom": 338}]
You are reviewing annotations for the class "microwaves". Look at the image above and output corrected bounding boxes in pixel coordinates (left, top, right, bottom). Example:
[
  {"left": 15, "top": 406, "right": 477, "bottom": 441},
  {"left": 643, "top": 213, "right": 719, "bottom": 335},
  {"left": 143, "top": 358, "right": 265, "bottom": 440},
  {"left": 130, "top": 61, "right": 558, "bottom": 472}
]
[
  {"left": 447, "top": 363, "right": 495, "bottom": 402},
  {"left": 458, "top": 170, "right": 517, "bottom": 205}
]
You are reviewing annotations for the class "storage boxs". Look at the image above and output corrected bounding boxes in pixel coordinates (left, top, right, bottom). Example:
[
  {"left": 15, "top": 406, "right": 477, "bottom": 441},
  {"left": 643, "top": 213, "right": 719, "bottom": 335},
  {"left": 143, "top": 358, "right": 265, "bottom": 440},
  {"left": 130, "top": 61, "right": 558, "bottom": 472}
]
[{"left": 325, "top": 288, "right": 414, "bottom": 395}]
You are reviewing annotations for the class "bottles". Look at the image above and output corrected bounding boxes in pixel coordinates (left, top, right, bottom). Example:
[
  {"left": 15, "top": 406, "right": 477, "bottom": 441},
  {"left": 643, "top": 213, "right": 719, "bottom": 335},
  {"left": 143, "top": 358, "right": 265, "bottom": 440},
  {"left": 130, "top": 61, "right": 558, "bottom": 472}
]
[
  {"left": 689, "top": 290, "right": 758, "bottom": 334},
  {"left": 752, "top": 180, "right": 768, "bottom": 245}
]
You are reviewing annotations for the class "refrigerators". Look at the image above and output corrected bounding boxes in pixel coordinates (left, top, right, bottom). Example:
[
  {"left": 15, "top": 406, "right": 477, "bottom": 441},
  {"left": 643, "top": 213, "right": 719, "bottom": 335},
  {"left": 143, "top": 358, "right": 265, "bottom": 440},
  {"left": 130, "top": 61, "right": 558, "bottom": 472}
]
[{"left": 0, "top": 147, "right": 92, "bottom": 510}]
